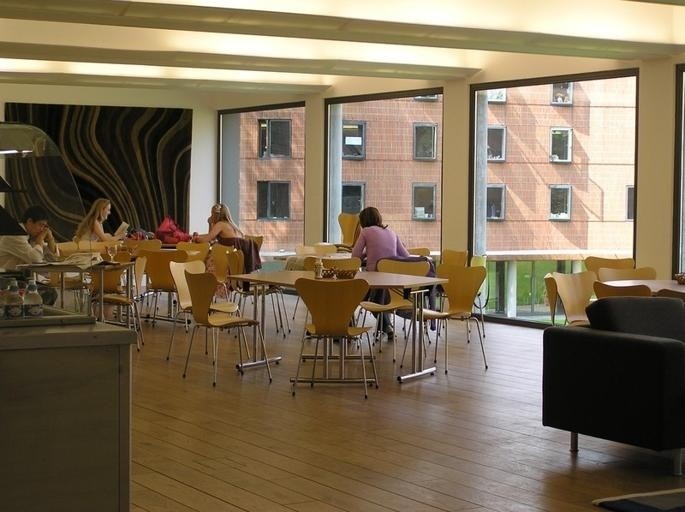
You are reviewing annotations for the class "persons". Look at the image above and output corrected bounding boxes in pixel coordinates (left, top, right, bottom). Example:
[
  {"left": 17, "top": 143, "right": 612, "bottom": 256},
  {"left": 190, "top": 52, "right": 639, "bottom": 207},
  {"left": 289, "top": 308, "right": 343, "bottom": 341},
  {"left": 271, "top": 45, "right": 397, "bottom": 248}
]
[
  {"left": 350, "top": 207, "right": 410, "bottom": 341},
  {"left": 0, "top": 205, "right": 62, "bottom": 306},
  {"left": 74, "top": 197, "right": 126, "bottom": 243},
  {"left": 192, "top": 202, "right": 244, "bottom": 251}
]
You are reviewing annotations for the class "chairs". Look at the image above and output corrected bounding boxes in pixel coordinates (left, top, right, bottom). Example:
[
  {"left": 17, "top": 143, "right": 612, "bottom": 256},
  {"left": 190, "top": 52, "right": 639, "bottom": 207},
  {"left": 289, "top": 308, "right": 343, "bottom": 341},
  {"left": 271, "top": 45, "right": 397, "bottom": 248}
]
[{"left": 543, "top": 255, "right": 685, "bottom": 477}]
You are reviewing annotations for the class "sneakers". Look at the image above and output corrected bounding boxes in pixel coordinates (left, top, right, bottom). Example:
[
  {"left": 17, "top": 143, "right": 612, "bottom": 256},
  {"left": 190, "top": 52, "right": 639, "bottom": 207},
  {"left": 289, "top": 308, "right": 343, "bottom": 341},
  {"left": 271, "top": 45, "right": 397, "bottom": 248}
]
[{"left": 375, "top": 325, "right": 395, "bottom": 340}]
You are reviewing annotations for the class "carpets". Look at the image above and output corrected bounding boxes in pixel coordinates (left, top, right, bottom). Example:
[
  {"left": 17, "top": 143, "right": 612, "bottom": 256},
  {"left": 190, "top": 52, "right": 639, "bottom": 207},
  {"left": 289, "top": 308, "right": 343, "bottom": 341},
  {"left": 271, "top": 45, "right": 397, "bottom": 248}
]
[{"left": 590, "top": 486, "right": 685, "bottom": 511}]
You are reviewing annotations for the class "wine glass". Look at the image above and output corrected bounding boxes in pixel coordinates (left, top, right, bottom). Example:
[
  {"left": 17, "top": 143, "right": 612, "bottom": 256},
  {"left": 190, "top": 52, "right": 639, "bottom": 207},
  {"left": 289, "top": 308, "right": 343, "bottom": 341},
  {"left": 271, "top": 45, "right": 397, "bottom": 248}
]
[{"left": 107, "top": 245, "right": 118, "bottom": 263}]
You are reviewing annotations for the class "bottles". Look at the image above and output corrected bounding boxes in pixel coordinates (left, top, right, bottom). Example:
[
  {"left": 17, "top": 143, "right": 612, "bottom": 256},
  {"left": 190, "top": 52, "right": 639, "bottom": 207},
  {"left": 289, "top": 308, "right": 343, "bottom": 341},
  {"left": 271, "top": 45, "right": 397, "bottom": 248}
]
[
  {"left": 0, "top": 278, "right": 44, "bottom": 320},
  {"left": 313, "top": 257, "right": 324, "bottom": 279},
  {"left": 193, "top": 232, "right": 199, "bottom": 243}
]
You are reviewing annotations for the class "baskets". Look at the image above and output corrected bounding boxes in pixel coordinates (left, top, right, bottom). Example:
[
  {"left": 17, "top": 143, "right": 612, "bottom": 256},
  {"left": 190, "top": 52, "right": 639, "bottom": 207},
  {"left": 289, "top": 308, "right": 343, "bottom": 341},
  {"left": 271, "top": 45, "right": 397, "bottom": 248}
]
[{"left": 335, "top": 268, "right": 359, "bottom": 279}]
